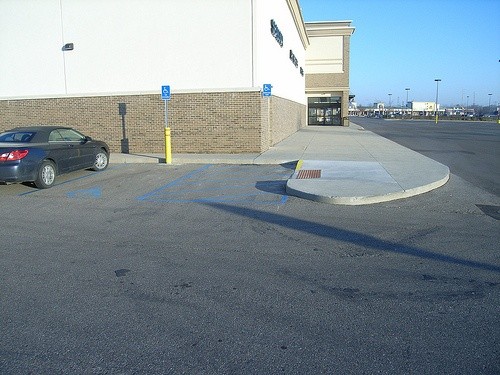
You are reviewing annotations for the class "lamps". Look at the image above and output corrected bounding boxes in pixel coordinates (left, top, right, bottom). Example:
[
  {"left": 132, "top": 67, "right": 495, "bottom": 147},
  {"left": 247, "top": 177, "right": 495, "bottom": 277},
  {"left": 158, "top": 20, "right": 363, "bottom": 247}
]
[{"left": 64, "top": 43, "right": 73, "bottom": 50}]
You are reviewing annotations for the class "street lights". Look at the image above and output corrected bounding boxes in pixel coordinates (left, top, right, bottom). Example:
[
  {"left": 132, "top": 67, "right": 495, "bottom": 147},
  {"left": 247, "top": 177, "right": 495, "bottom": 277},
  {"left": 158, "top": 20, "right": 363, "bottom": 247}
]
[
  {"left": 487, "top": 93, "right": 492, "bottom": 115},
  {"left": 466, "top": 96, "right": 470, "bottom": 114},
  {"left": 388, "top": 93, "right": 393, "bottom": 109},
  {"left": 404, "top": 87, "right": 411, "bottom": 103},
  {"left": 434, "top": 78, "right": 443, "bottom": 125}
]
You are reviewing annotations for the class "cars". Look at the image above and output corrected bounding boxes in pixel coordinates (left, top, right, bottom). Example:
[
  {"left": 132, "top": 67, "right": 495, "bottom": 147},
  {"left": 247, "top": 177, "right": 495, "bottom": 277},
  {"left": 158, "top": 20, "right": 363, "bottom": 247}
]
[{"left": 0, "top": 124, "right": 111, "bottom": 189}]
